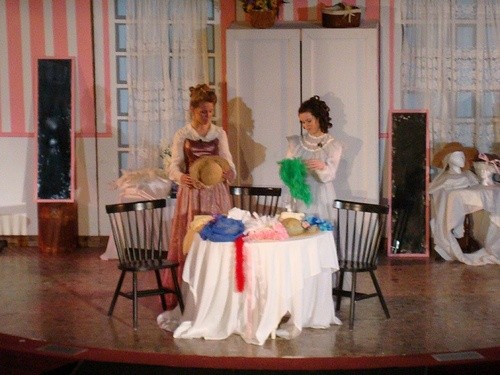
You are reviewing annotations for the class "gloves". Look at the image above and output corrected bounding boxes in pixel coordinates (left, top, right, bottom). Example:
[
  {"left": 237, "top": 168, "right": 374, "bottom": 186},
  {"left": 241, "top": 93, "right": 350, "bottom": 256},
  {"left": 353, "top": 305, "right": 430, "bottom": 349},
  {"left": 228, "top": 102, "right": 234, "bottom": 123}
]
[{"left": 280, "top": 218, "right": 319, "bottom": 238}]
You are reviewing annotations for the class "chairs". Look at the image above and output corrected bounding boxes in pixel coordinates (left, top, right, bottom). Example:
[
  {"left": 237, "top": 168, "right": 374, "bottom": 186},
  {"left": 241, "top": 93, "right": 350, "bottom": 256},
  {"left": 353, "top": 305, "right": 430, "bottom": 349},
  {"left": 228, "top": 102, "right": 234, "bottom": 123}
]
[
  {"left": 332, "top": 199, "right": 391, "bottom": 329},
  {"left": 106, "top": 199, "right": 185, "bottom": 330},
  {"left": 229, "top": 185, "right": 281, "bottom": 217}
]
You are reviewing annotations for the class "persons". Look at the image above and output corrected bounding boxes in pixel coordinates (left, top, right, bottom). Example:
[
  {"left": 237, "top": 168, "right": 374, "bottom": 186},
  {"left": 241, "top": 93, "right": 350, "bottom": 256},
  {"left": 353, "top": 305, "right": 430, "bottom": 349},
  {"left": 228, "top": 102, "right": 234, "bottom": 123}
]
[
  {"left": 433, "top": 142, "right": 479, "bottom": 190},
  {"left": 473, "top": 153, "right": 500, "bottom": 186},
  {"left": 161, "top": 83, "right": 237, "bottom": 310},
  {"left": 276, "top": 95, "right": 343, "bottom": 227}
]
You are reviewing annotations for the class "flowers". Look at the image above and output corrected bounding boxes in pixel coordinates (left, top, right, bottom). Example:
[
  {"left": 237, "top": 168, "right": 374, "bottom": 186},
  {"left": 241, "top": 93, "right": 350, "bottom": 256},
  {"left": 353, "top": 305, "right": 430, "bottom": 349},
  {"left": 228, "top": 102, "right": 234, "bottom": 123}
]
[{"left": 241, "top": 1, "right": 289, "bottom": 12}]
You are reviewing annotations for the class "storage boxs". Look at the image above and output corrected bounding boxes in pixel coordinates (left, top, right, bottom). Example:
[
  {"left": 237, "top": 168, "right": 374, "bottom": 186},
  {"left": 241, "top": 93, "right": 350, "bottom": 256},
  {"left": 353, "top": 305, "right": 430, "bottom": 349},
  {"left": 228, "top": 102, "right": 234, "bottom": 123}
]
[{"left": 321, "top": 3, "right": 361, "bottom": 28}]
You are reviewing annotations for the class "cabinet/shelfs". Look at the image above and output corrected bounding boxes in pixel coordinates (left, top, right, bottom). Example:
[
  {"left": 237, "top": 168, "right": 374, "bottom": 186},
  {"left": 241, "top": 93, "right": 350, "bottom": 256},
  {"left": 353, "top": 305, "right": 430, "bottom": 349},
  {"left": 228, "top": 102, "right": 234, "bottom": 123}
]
[{"left": 226, "top": 20, "right": 380, "bottom": 264}]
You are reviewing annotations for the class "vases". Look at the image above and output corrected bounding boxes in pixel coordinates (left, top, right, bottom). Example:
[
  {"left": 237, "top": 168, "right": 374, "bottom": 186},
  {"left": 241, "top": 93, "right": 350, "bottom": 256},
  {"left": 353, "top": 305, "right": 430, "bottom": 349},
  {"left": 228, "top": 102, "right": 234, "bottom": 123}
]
[{"left": 247, "top": 12, "right": 279, "bottom": 29}]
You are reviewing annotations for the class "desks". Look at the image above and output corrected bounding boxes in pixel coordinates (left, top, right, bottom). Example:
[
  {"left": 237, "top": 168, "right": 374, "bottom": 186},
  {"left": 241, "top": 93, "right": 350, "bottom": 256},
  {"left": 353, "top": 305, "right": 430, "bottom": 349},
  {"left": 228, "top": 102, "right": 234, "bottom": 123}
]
[
  {"left": 194, "top": 229, "right": 332, "bottom": 328},
  {"left": 447, "top": 184, "right": 499, "bottom": 253}
]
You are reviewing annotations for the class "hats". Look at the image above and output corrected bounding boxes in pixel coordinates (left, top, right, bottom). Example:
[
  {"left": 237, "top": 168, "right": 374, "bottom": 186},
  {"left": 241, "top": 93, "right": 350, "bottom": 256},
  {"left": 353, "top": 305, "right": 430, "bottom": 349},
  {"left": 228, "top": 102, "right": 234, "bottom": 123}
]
[
  {"left": 472, "top": 153, "right": 500, "bottom": 167},
  {"left": 189, "top": 155, "right": 230, "bottom": 190},
  {"left": 432, "top": 142, "right": 479, "bottom": 170}
]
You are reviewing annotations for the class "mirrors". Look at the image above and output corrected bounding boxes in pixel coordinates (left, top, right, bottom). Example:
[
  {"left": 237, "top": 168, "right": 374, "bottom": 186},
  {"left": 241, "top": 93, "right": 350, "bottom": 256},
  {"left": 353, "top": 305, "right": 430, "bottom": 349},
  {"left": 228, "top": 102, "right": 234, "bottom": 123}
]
[
  {"left": 33, "top": 55, "right": 75, "bottom": 204},
  {"left": 388, "top": 110, "right": 430, "bottom": 257}
]
